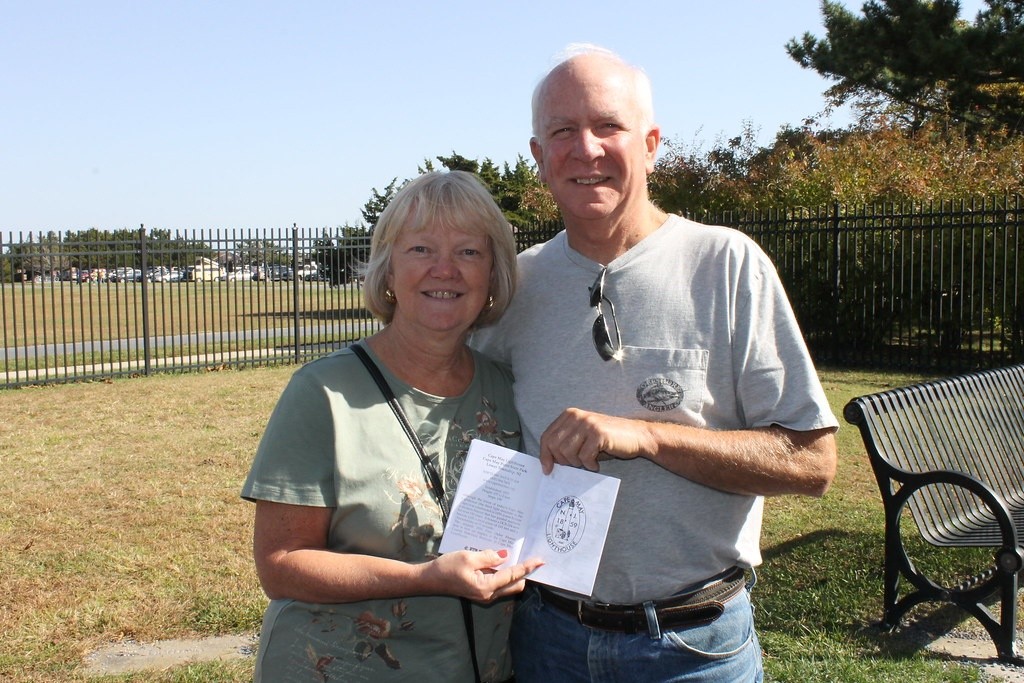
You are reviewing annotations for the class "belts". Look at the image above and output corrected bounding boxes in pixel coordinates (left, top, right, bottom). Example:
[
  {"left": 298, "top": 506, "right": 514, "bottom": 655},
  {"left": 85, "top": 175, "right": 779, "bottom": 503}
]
[{"left": 530, "top": 569, "right": 749, "bottom": 635}]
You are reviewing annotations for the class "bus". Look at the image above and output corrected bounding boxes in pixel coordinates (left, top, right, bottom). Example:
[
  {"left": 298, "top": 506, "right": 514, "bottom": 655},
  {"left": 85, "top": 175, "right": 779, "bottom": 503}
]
[{"left": 181, "top": 256, "right": 221, "bottom": 283}]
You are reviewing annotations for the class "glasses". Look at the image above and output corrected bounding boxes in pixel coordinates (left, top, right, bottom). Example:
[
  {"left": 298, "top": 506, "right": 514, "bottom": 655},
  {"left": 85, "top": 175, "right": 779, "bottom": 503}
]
[{"left": 586, "top": 268, "right": 623, "bottom": 362}]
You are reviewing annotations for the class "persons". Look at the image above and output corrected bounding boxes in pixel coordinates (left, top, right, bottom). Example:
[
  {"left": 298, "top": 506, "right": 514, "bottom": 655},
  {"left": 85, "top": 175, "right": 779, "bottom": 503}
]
[
  {"left": 466, "top": 51, "right": 838, "bottom": 683},
  {"left": 240, "top": 172, "right": 545, "bottom": 683}
]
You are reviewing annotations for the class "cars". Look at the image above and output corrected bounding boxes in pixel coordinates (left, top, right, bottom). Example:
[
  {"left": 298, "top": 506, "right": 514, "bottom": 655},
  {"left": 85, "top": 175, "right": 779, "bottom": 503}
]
[
  {"left": 57, "top": 267, "right": 184, "bottom": 283},
  {"left": 221, "top": 262, "right": 320, "bottom": 281}
]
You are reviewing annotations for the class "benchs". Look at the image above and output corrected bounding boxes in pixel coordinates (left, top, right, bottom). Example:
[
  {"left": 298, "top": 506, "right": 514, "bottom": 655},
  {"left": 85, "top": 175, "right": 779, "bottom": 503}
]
[{"left": 842, "top": 361, "right": 1024, "bottom": 668}]
